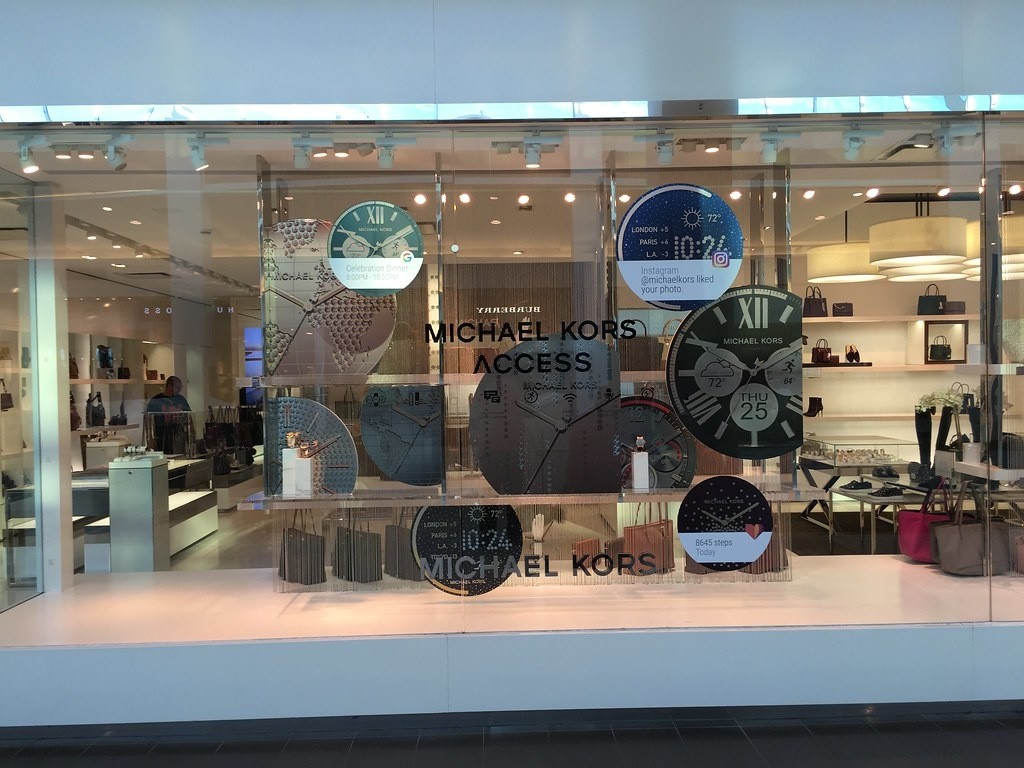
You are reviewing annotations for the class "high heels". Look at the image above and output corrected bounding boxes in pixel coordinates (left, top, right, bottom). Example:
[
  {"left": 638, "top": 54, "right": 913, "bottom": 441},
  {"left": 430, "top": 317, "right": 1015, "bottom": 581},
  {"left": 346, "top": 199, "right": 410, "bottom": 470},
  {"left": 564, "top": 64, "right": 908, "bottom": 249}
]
[{"left": 804, "top": 397, "right": 823, "bottom": 417}]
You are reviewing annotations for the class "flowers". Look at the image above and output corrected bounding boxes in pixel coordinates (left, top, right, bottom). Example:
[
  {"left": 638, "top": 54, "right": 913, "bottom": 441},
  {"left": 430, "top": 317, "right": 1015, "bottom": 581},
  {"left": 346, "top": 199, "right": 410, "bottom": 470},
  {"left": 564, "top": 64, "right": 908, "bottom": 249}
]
[{"left": 915, "top": 389, "right": 964, "bottom": 450}]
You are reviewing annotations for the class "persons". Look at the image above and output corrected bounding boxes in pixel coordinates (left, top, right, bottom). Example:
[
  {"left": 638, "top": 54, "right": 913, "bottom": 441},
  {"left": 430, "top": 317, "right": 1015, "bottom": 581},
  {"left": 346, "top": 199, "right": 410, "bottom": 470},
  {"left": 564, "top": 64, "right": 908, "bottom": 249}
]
[{"left": 144, "top": 376, "right": 196, "bottom": 456}]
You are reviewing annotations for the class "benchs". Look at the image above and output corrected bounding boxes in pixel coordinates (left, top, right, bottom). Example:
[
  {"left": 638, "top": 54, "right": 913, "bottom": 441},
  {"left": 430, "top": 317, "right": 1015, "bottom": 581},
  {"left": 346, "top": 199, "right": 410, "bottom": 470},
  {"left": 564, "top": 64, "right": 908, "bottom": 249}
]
[
  {"left": 214, "top": 454, "right": 265, "bottom": 512},
  {"left": 2, "top": 490, "right": 218, "bottom": 587}
]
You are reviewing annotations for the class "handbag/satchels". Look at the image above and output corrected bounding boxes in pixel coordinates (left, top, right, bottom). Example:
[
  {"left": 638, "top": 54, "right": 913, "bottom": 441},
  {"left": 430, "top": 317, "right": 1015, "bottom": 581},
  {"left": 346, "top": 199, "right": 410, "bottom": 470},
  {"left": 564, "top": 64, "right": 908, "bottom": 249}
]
[
  {"left": 917, "top": 283, "right": 946, "bottom": 314},
  {"left": 897, "top": 475, "right": 1015, "bottom": 576},
  {"left": 143, "top": 404, "right": 255, "bottom": 475},
  {"left": 147, "top": 369, "right": 158, "bottom": 381},
  {"left": 802, "top": 286, "right": 828, "bottom": 317},
  {"left": 70, "top": 392, "right": 81, "bottom": 431},
  {"left": 118, "top": 367, "right": 131, "bottom": 379},
  {"left": 952, "top": 383, "right": 974, "bottom": 412},
  {"left": 811, "top": 339, "right": 831, "bottom": 363},
  {"left": 96, "top": 345, "right": 114, "bottom": 369},
  {"left": 69, "top": 353, "right": 79, "bottom": 380},
  {"left": 929, "top": 335, "right": 951, "bottom": 359},
  {"left": 108, "top": 414, "right": 127, "bottom": 425},
  {"left": 0, "top": 378, "right": 13, "bottom": 410},
  {"left": 86, "top": 392, "right": 105, "bottom": 426},
  {"left": 833, "top": 302, "right": 853, "bottom": 316}
]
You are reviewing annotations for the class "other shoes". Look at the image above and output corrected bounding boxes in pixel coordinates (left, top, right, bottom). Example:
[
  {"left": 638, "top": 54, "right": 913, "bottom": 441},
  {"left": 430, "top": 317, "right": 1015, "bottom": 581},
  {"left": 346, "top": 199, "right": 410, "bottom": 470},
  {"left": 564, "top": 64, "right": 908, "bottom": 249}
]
[
  {"left": 838, "top": 462, "right": 957, "bottom": 498},
  {"left": 845, "top": 345, "right": 860, "bottom": 363}
]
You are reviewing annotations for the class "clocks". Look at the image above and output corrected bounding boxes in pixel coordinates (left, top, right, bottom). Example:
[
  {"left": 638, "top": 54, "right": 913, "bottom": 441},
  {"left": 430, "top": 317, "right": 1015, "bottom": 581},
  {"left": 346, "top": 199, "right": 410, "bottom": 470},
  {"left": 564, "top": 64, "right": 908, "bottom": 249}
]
[
  {"left": 677, "top": 475, "right": 773, "bottom": 572},
  {"left": 260, "top": 202, "right": 425, "bottom": 375},
  {"left": 470, "top": 182, "right": 805, "bottom": 493},
  {"left": 267, "top": 384, "right": 443, "bottom": 493}
]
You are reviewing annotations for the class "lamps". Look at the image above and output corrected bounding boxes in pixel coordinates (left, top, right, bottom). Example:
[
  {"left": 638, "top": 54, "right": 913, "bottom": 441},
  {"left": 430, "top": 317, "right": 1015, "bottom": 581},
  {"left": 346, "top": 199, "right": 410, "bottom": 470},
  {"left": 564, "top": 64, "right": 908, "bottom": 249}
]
[
  {"left": 496, "top": 131, "right": 542, "bottom": 168},
  {"left": 292, "top": 131, "right": 396, "bottom": 169},
  {"left": 843, "top": 125, "right": 865, "bottom": 161},
  {"left": 759, "top": 126, "right": 779, "bottom": 162},
  {"left": 13, "top": 130, "right": 208, "bottom": 173},
  {"left": 914, "top": 123, "right": 975, "bottom": 156},
  {"left": 807, "top": 193, "right": 1024, "bottom": 284},
  {"left": 654, "top": 129, "right": 741, "bottom": 164}
]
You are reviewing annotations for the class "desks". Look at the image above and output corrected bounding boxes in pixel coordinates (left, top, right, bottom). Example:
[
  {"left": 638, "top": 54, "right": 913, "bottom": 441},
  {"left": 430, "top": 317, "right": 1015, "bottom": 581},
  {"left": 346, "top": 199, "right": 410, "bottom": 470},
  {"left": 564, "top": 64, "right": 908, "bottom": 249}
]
[{"left": 828, "top": 474, "right": 1024, "bottom": 555}]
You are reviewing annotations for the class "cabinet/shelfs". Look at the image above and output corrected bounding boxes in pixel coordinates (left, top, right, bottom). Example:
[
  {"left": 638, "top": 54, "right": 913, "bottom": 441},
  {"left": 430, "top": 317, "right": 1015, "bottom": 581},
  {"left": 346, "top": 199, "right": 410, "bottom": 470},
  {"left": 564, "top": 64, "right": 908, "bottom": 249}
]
[
  {"left": 237, "top": 367, "right": 825, "bottom": 510},
  {"left": 70, "top": 379, "right": 167, "bottom": 435},
  {"left": 0, "top": 368, "right": 35, "bottom": 542},
  {"left": 802, "top": 314, "right": 1023, "bottom": 419},
  {"left": 954, "top": 364, "right": 1024, "bottom": 481}
]
